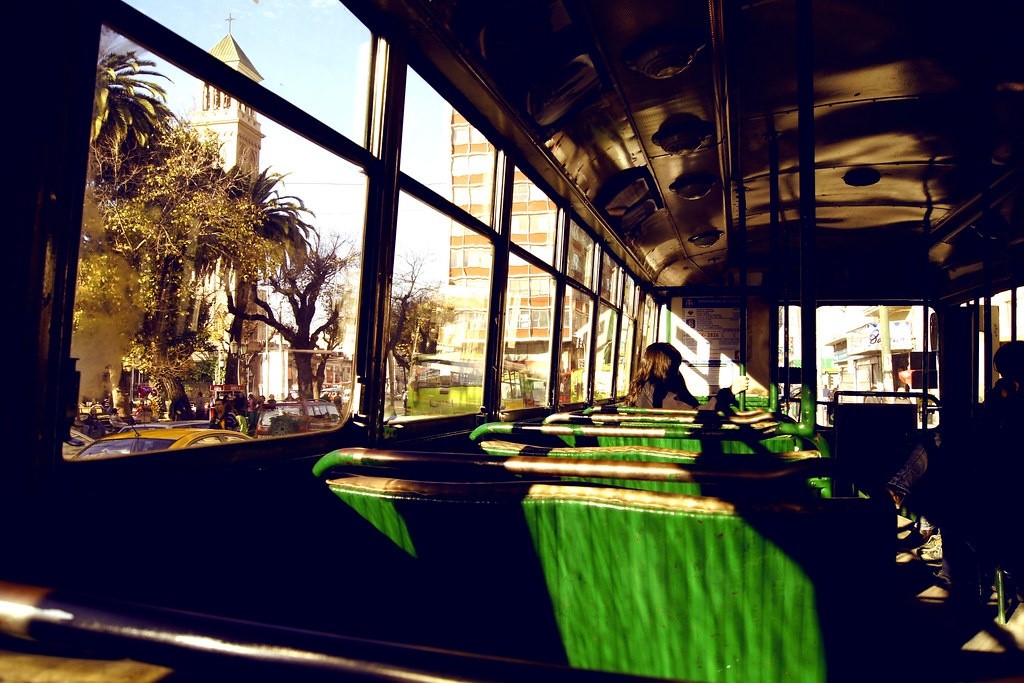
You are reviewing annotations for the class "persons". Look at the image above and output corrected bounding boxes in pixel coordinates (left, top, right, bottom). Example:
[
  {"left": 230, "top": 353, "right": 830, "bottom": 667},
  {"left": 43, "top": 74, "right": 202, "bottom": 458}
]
[
  {"left": 815, "top": 337, "right": 1024, "bottom": 591},
  {"left": 625, "top": 342, "right": 749, "bottom": 411},
  {"left": 129, "top": 382, "right": 343, "bottom": 421},
  {"left": 87, "top": 407, "right": 110, "bottom": 435},
  {"left": 109, "top": 408, "right": 129, "bottom": 428},
  {"left": 210, "top": 399, "right": 241, "bottom": 432},
  {"left": 231, "top": 409, "right": 248, "bottom": 434}
]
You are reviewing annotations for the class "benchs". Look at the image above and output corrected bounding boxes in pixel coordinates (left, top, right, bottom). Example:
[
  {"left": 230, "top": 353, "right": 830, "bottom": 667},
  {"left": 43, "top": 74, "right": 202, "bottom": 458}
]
[
  {"left": 583, "top": 405, "right": 797, "bottom": 430},
  {"left": 309, "top": 445, "right": 898, "bottom": 683},
  {"left": 540, "top": 412, "right": 804, "bottom": 454},
  {"left": 459, "top": 421, "right": 830, "bottom": 500}
]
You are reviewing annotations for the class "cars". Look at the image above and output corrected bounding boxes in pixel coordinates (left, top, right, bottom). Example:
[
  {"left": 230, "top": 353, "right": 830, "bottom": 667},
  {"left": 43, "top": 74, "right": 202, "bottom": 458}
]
[
  {"left": 117, "top": 420, "right": 211, "bottom": 434},
  {"left": 69, "top": 428, "right": 253, "bottom": 461},
  {"left": 62, "top": 428, "right": 95, "bottom": 460},
  {"left": 526, "top": 378, "right": 547, "bottom": 408}
]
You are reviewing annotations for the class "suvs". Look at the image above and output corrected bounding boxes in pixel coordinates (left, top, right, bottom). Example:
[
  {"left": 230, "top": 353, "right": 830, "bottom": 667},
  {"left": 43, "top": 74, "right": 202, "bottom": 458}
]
[{"left": 254, "top": 398, "right": 343, "bottom": 438}]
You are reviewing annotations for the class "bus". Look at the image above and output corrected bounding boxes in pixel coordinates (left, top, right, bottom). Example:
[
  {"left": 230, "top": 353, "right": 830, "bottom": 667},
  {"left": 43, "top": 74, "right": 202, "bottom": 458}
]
[{"left": 407, "top": 353, "right": 528, "bottom": 417}]
